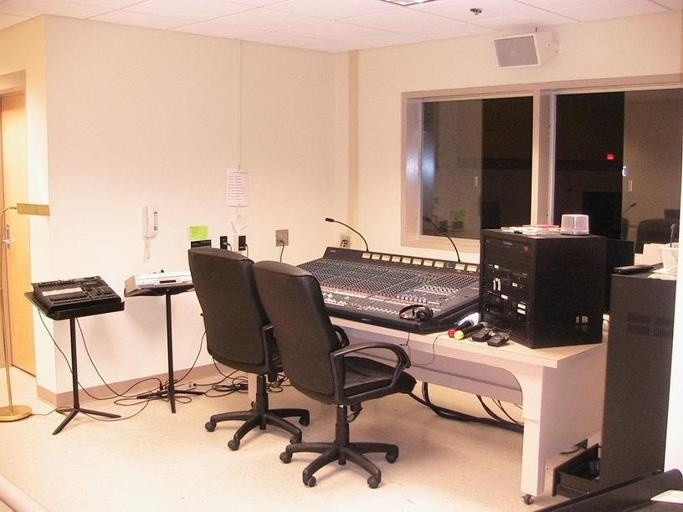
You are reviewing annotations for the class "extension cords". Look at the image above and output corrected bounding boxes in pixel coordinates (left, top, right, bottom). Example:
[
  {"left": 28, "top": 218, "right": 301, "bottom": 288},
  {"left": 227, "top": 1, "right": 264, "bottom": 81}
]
[{"left": 152, "top": 381, "right": 197, "bottom": 396}]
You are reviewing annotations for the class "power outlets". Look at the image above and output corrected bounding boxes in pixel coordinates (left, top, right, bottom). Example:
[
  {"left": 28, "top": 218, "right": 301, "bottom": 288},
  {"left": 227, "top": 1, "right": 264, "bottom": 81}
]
[
  {"left": 221, "top": 236, "right": 227, "bottom": 249},
  {"left": 239, "top": 236, "right": 246, "bottom": 251}
]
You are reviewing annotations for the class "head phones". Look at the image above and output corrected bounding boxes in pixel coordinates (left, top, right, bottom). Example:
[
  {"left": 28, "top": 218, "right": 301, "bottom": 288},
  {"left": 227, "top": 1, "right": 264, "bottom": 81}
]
[{"left": 399, "top": 303, "right": 434, "bottom": 320}]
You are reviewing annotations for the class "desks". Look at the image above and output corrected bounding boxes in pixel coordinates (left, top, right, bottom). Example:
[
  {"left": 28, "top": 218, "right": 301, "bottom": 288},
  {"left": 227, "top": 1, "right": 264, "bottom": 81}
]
[{"left": 326, "top": 315, "right": 607, "bottom": 505}]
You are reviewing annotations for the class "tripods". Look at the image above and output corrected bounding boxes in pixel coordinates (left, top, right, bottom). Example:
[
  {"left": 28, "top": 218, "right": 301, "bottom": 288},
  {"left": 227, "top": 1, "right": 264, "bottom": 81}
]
[
  {"left": 137, "top": 297, "right": 203, "bottom": 413},
  {"left": 51, "top": 321, "right": 121, "bottom": 435}
]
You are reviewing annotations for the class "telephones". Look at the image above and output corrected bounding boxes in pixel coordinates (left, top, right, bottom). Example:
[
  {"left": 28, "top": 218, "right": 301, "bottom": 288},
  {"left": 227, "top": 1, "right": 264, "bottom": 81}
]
[{"left": 145, "top": 206, "right": 159, "bottom": 239}]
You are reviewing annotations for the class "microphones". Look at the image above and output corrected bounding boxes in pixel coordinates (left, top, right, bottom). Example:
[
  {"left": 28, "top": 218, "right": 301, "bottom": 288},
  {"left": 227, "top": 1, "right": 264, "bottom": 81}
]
[
  {"left": 621, "top": 201, "right": 636, "bottom": 220},
  {"left": 423, "top": 215, "right": 459, "bottom": 262},
  {"left": 325, "top": 217, "right": 368, "bottom": 251},
  {"left": 455, "top": 322, "right": 487, "bottom": 341},
  {"left": 449, "top": 319, "right": 474, "bottom": 338}
]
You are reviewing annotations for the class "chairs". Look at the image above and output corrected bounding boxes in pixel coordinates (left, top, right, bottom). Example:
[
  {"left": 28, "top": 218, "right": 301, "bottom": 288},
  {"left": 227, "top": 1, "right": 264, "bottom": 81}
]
[
  {"left": 253, "top": 261, "right": 416, "bottom": 487},
  {"left": 634, "top": 218, "right": 672, "bottom": 253},
  {"left": 189, "top": 247, "right": 311, "bottom": 451},
  {"left": 621, "top": 217, "right": 629, "bottom": 241}
]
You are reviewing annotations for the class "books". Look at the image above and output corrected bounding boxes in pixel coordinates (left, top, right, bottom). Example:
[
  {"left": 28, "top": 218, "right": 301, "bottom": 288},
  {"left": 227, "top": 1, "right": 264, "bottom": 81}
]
[
  {"left": 648, "top": 265, "right": 676, "bottom": 280},
  {"left": 499, "top": 222, "right": 561, "bottom": 236}
]
